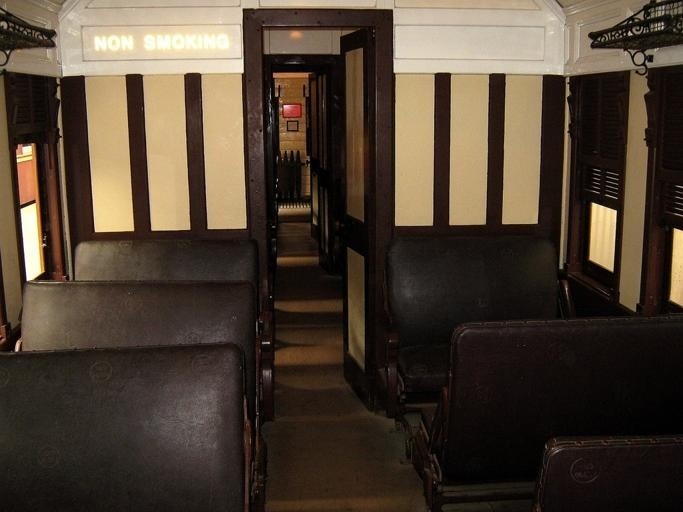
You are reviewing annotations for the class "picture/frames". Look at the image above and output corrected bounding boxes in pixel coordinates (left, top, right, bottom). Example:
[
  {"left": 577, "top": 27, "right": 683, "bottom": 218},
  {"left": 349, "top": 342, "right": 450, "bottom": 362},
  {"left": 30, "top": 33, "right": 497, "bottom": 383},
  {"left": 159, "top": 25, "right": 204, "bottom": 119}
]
[
  {"left": 282, "top": 103, "right": 302, "bottom": 118},
  {"left": 286, "top": 120, "right": 299, "bottom": 131}
]
